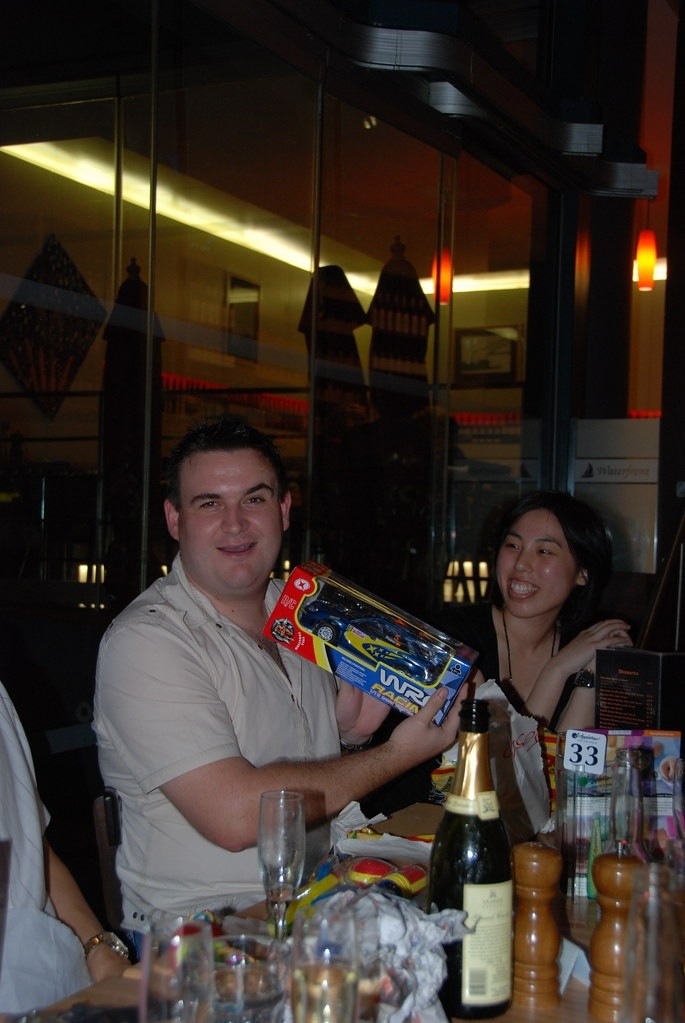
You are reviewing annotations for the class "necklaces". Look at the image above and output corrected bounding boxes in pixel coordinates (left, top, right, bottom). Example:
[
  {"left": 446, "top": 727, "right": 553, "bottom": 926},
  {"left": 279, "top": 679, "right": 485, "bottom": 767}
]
[{"left": 501, "top": 607, "right": 556, "bottom": 687}]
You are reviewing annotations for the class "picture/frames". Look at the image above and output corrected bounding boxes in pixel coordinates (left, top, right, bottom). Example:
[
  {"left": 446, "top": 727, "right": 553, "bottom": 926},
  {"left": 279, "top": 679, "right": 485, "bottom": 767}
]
[
  {"left": 221, "top": 273, "right": 261, "bottom": 367},
  {"left": 448, "top": 324, "right": 521, "bottom": 386}
]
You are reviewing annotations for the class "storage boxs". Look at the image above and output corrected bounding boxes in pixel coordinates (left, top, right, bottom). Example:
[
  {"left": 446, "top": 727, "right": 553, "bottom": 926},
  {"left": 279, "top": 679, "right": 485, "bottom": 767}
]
[{"left": 262, "top": 558, "right": 480, "bottom": 728}]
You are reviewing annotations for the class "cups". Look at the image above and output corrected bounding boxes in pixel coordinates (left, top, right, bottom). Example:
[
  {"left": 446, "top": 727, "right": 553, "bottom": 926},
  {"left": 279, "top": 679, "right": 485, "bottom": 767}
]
[
  {"left": 141, "top": 916, "right": 216, "bottom": 1023},
  {"left": 288, "top": 907, "right": 358, "bottom": 1023},
  {"left": 212, "top": 933, "right": 292, "bottom": 1023}
]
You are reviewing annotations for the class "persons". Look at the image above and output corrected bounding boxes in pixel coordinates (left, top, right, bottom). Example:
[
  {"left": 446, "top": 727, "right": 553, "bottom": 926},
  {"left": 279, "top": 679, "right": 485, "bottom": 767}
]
[
  {"left": 387, "top": 491, "right": 637, "bottom": 812},
  {"left": 0, "top": 684, "right": 182, "bottom": 1023},
  {"left": 89, "top": 417, "right": 472, "bottom": 973}
]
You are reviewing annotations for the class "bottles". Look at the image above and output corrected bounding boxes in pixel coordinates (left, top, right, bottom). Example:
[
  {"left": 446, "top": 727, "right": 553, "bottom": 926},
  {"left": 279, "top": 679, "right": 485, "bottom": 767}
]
[
  {"left": 610, "top": 749, "right": 648, "bottom": 875},
  {"left": 429, "top": 697, "right": 516, "bottom": 1021}
]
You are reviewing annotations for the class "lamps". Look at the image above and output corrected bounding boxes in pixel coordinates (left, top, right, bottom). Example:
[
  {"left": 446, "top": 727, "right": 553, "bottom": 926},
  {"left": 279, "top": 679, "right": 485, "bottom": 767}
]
[
  {"left": 431, "top": 155, "right": 455, "bottom": 307},
  {"left": 636, "top": 197, "right": 657, "bottom": 291}
]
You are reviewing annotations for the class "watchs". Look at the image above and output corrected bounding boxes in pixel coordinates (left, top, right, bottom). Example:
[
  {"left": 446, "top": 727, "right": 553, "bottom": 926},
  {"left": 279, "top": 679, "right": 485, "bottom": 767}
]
[
  {"left": 81, "top": 932, "right": 132, "bottom": 964},
  {"left": 569, "top": 666, "right": 595, "bottom": 691}
]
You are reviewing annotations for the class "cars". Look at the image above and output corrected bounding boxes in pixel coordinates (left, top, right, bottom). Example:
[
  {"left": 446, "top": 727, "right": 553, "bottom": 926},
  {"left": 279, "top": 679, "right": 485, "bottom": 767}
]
[{"left": 301, "top": 603, "right": 448, "bottom": 685}]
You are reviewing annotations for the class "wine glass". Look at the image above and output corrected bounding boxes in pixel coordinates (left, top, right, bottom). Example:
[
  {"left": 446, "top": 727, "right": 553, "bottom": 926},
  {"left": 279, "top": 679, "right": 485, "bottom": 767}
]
[{"left": 256, "top": 791, "right": 307, "bottom": 942}]
